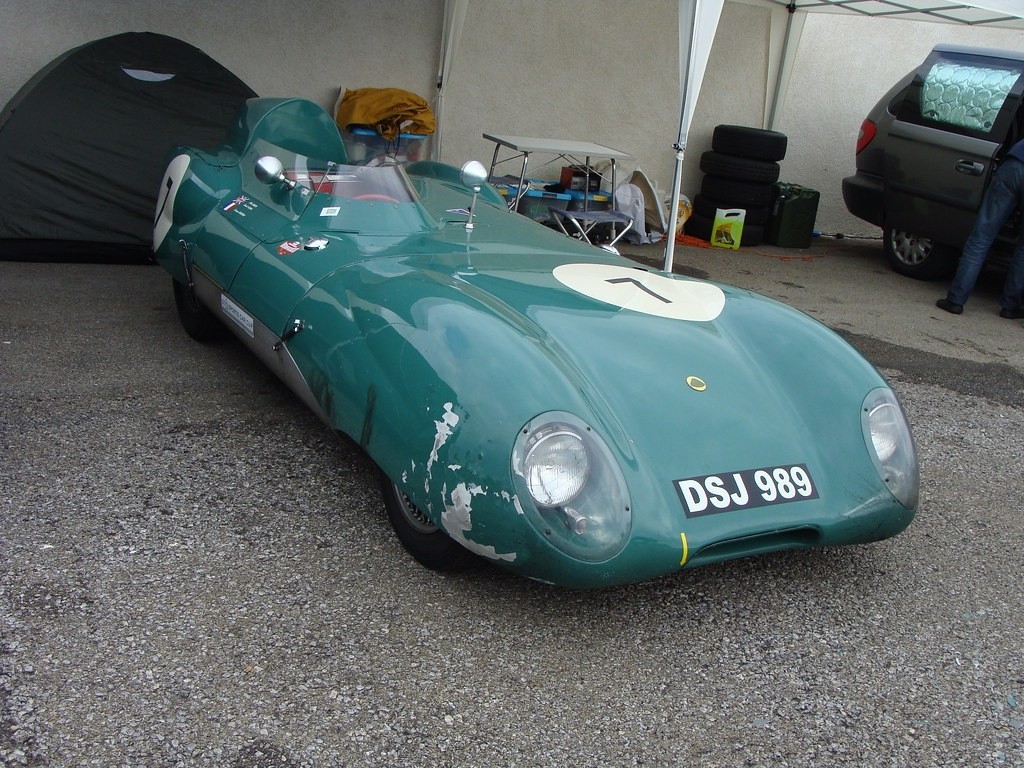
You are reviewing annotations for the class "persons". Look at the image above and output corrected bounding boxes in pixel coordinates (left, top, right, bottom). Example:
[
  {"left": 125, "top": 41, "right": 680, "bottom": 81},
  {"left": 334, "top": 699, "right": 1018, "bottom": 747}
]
[{"left": 936, "top": 138, "right": 1024, "bottom": 320}]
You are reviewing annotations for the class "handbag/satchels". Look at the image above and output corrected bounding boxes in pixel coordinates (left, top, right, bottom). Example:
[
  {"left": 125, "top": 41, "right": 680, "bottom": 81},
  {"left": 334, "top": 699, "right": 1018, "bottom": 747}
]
[{"left": 710, "top": 208, "right": 746, "bottom": 250}]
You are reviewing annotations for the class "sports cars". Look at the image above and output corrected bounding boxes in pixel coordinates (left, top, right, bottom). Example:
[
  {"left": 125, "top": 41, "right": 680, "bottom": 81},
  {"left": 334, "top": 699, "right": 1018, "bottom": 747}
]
[{"left": 151, "top": 96, "right": 920, "bottom": 588}]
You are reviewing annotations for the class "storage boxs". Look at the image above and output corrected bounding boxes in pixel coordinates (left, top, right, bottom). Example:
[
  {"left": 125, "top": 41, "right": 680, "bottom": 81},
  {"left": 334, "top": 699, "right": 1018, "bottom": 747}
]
[
  {"left": 550, "top": 181, "right": 612, "bottom": 226},
  {"left": 523, "top": 180, "right": 572, "bottom": 223},
  {"left": 489, "top": 176, "right": 522, "bottom": 211}
]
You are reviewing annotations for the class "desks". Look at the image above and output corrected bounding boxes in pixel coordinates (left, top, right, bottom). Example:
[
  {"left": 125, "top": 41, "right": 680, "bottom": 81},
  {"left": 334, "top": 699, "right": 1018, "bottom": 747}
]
[
  {"left": 352, "top": 128, "right": 432, "bottom": 162},
  {"left": 479, "top": 132, "right": 633, "bottom": 242}
]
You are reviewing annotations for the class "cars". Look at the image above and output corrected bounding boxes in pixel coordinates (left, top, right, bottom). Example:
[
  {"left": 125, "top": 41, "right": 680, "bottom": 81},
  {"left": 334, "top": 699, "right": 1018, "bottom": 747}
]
[{"left": 843, "top": 44, "right": 1024, "bottom": 281}]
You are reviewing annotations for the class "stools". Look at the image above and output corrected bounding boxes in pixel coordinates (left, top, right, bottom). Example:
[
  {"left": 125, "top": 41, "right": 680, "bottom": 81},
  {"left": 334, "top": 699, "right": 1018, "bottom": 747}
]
[{"left": 547, "top": 206, "right": 634, "bottom": 247}]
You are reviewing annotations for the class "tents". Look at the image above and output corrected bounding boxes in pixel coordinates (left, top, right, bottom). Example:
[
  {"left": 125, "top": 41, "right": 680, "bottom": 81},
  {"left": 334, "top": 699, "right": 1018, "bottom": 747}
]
[{"left": 425, "top": 0, "right": 1024, "bottom": 272}]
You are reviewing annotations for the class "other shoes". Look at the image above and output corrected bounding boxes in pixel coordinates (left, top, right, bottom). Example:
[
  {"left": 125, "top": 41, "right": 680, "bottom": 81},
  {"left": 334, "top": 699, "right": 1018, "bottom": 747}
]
[
  {"left": 1000, "top": 308, "right": 1024, "bottom": 319},
  {"left": 937, "top": 299, "right": 964, "bottom": 314}
]
